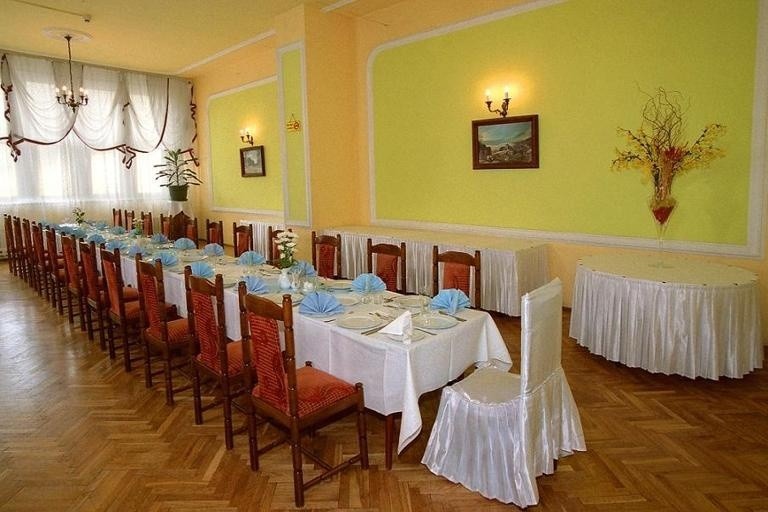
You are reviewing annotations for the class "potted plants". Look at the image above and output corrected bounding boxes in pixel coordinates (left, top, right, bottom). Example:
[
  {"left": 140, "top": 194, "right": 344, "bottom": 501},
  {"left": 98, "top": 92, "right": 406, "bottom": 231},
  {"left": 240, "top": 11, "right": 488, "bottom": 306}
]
[{"left": 153, "top": 148, "right": 204, "bottom": 201}]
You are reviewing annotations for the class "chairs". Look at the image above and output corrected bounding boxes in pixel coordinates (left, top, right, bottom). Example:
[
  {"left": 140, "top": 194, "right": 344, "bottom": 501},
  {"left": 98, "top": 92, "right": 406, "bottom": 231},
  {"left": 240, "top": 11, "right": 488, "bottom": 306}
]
[
  {"left": 23, "top": 219, "right": 61, "bottom": 289},
  {"left": 45, "top": 230, "right": 82, "bottom": 314},
  {"left": 3, "top": 214, "right": 34, "bottom": 276},
  {"left": 141, "top": 212, "right": 153, "bottom": 236},
  {"left": 133, "top": 253, "right": 200, "bottom": 406},
  {"left": 125, "top": 210, "right": 135, "bottom": 231},
  {"left": 183, "top": 266, "right": 287, "bottom": 450},
  {"left": 61, "top": 231, "right": 102, "bottom": 333},
  {"left": 238, "top": 281, "right": 370, "bottom": 508},
  {"left": 113, "top": 208, "right": 123, "bottom": 228},
  {"left": 233, "top": 222, "right": 253, "bottom": 258},
  {"left": 99, "top": 245, "right": 177, "bottom": 373},
  {"left": 368, "top": 238, "right": 406, "bottom": 295},
  {"left": 267, "top": 226, "right": 292, "bottom": 266},
  {"left": 206, "top": 219, "right": 224, "bottom": 253},
  {"left": 79, "top": 242, "right": 137, "bottom": 340},
  {"left": 431, "top": 245, "right": 481, "bottom": 312},
  {"left": 32, "top": 222, "right": 64, "bottom": 302},
  {"left": 421, "top": 279, "right": 586, "bottom": 508},
  {"left": 160, "top": 211, "right": 199, "bottom": 249},
  {"left": 13, "top": 217, "right": 45, "bottom": 282},
  {"left": 311, "top": 232, "right": 342, "bottom": 280}
]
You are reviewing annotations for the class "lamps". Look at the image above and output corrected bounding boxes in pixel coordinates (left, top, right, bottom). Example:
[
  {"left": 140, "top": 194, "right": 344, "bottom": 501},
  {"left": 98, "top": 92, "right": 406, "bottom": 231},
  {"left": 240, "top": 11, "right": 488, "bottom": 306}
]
[
  {"left": 240, "top": 127, "right": 254, "bottom": 146},
  {"left": 485, "top": 85, "right": 515, "bottom": 118}
]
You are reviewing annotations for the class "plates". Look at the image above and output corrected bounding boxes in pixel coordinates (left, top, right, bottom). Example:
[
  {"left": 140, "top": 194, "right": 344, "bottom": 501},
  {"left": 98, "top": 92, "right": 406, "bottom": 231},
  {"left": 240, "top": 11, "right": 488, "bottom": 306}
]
[
  {"left": 412, "top": 314, "right": 457, "bottom": 329},
  {"left": 272, "top": 293, "right": 304, "bottom": 303},
  {"left": 324, "top": 283, "right": 352, "bottom": 290},
  {"left": 388, "top": 332, "right": 426, "bottom": 343},
  {"left": 337, "top": 297, "right": 359, "bottom": 307},
  {"left": 336, "top": 314, "right": 383, "bottom": 329},
  {"left": 209, "top": 277, "right": 239, "bottom": 288},
  {"left": 398, "top": 296, "right": 431, "bottom": 307}
]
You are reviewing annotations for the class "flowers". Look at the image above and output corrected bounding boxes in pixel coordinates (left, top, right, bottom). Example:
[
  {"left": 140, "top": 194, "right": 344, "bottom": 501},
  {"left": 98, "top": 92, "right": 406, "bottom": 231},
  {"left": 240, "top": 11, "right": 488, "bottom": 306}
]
[
  {"left": 72, "top": 208, "right": 88, "bottom": 227},
  {"left": 132, "top": 218, "right": 143, "bottom": 234},
  {"left": 274, "top": 230, "right": 299, "bottom": 272},
  {"left": 608, "top": 82, "right": 729, "bottom": 236}
]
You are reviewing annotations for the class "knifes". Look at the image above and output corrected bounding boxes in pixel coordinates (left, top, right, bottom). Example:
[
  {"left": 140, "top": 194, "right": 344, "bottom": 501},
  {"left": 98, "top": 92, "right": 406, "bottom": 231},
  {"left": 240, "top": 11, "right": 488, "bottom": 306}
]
[
  {"left": 324, "top": 311, "right": 354, "bottom": 322},
  {"left": 439, "top": 311, "right": 466, "bottom": 322},
  {"left": 413, "top": 326, "right": 437, "bottom": 336}
]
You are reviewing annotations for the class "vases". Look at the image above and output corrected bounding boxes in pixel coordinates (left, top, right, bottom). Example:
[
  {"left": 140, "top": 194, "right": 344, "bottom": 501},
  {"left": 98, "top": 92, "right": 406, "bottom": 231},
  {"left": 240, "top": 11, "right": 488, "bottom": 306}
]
[
  {"left": 280, "top": 268, "right": 292, "bottom": 290},
  {"left": 646, "top": 198, "right": 678, "bottom": 271},
  {"left": 135, "top": 234, "right": 144, "bottom": 248}
]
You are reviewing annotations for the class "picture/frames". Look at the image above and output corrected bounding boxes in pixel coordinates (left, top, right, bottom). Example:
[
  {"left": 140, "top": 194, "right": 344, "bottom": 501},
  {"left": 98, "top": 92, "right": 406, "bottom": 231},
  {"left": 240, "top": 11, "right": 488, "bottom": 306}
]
[
  {"left": 239, "top": 145, "right": 265, "bottom": 176},
  {"left": 472, "top": 115, "right": 539, "bottom": 172}
]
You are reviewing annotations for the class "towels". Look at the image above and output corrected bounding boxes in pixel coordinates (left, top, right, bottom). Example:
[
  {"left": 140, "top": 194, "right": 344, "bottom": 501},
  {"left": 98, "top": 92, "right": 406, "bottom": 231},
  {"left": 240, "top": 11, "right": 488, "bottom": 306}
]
[
  {"left": 431, "top": 287, "right": 471, "bottom": 315},
  {"left": 110, "top": 226, "right": 125, "bottom": 234},
  {"left": 127, "top": 229, "right": 136, "bottom": 238},
  {"left": 58, "top": 226, "right": 72, "bottom": 234},
  {"left": 240, "top": 275, "right": 268, "bottom": 293},
  {"left": 190, "top": 262, "right": 214, "bottom": 278},
  {"left": 154, "top": 252, "right": 177, "bottom": 265},
  {"left": 296, "top": 260, "right": 316, "bottom": 277},
  {"left": 299, "top": 291, "right": 344, "bottom": 314},
  {"left": 238, "top": 251, "right": 264, "bottom": 265},
  {"left": 152, "top": 233, "right": 167, "bottom": 242},
  {"left": 108, "top": 239, "right": 125, "bottom": 249},
  {"left": 95, "top": 223, "right": 107, "bottom": 230},
  {"left": 203, "top": 243, "right": 224, "bottom": 256},
  {"left": 174, "top": 237, "right": 196, "bottom": 249},
  {"left": 378, "top": 311, "right": 412, "bottom": 335},
  {"left": 128, "top": 243, "right": 145, "bottom": 255},
  {"left": 71, "top": 228, "right": 84, "bottom": 236},
  {"left": 88, "top": 234, "right": 104, "bottom": 244},
  {"left": 350, "top": 273, "right": 387, "bottom": 293}
]
